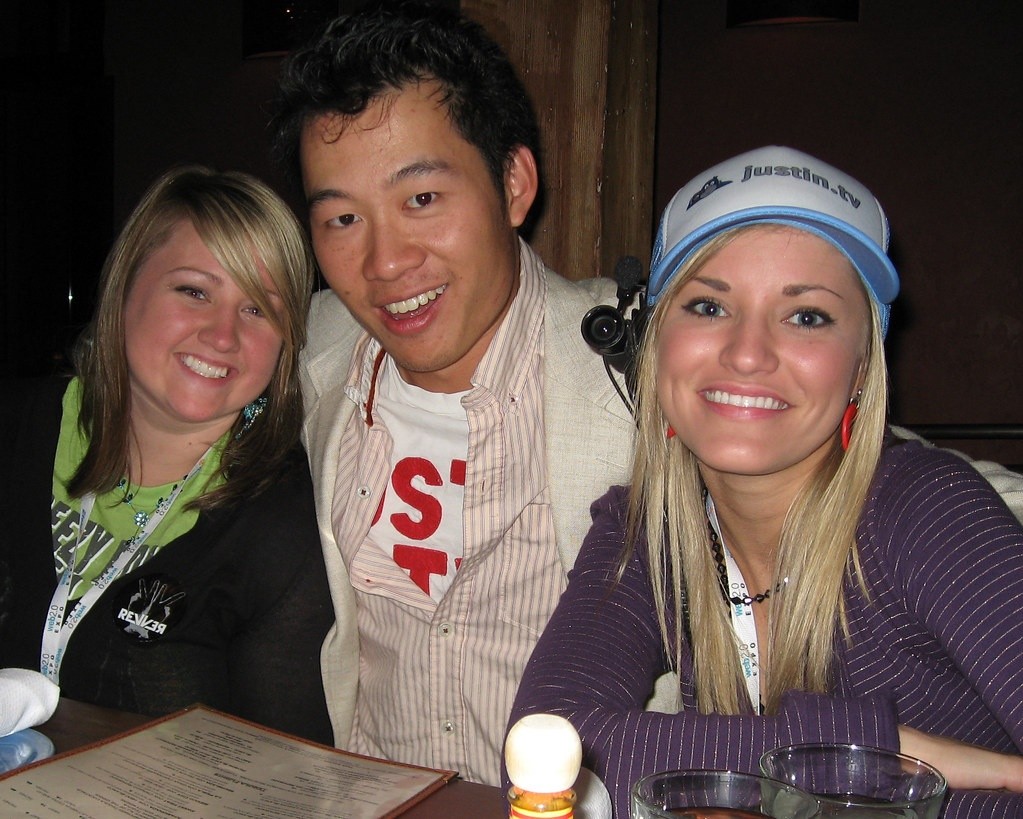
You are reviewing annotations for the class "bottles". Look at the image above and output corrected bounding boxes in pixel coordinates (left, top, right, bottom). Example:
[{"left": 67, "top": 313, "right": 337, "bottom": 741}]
[{"left": 506, "top": 714, "right": 583, "bottom": 819}]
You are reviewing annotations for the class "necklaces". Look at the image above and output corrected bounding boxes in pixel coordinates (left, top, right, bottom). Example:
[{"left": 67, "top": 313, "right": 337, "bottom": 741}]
[
  {"left": 698, "top": 483, "right": 787, "bottom": 605},
  {"left": 118, "top": 474, "right": 187, "bottom": 546}
]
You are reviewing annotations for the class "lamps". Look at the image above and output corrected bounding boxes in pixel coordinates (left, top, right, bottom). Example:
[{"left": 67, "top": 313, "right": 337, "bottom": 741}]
[
  {"left": 726, "top": 0, "right": 859, "bottom": 30},
  {"left": 241, "top": 0, "right": 339, "bottom": 60}
]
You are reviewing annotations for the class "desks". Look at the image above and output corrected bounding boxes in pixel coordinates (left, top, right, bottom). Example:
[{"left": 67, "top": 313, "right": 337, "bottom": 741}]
[{"left": 33, "top": 697, "right": 506, "bottom": 819}]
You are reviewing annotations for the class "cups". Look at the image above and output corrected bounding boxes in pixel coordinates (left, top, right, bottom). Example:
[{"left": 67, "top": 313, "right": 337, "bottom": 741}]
[
  {"left": 631, "top": 769, "right": 821, "bottom": 819},
  {"left": 759, "top": 742, "right": 948, "bottom": 819}
]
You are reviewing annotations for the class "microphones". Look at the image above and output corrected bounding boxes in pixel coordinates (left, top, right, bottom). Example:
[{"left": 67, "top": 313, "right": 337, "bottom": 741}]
[{"left": 614, "top": 255, "right": 642, "bottom": 313}]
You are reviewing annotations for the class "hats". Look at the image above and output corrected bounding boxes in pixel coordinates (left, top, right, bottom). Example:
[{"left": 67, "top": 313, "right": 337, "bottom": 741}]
[{"left": 647, "top": 146, "right": 899, "bottom": 343}]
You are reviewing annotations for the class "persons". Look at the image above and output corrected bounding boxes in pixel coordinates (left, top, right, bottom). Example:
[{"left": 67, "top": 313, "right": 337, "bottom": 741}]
[
  {"left": 0, "top": 166, "right": 354, "bottom": 745},
  {"left": 501, "top": 142, "right": 1023, "bottom": 819},
  {"left": 275, "top": 7, "right": 1023, "bottom": 819}
]
